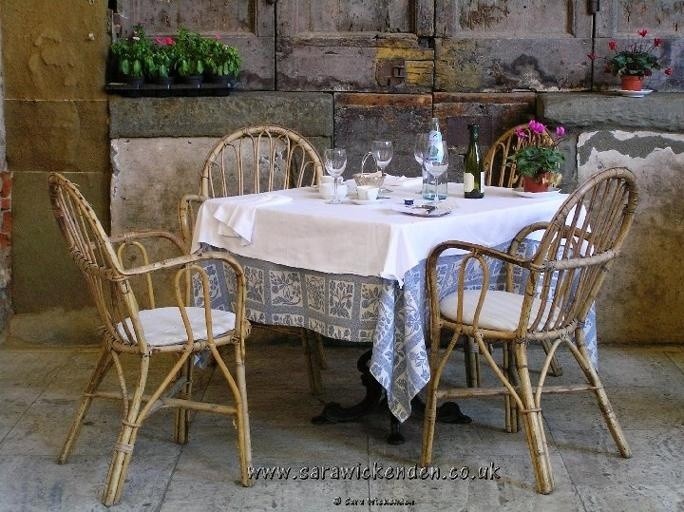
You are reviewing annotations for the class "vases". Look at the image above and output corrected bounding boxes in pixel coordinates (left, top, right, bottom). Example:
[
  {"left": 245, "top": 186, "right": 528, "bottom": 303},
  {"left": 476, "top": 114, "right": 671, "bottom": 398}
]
[{"left": 621, "top": 76, "right": 641, "bottom": 91}]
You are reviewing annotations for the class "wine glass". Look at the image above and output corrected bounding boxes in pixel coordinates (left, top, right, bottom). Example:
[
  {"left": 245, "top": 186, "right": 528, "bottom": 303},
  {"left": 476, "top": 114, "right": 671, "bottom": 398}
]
[
  {"left": 411, "top": 131, "right": 450, "bottom": 206},
  {"left": 373, "top": 140, "right": 393, "bottom": 193},
  {"left": 324, "top": 147, "right": 346, "bottom": 203}
]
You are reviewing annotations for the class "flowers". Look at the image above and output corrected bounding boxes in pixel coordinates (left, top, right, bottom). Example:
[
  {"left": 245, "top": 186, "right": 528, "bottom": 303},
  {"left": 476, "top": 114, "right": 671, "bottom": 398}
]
[
  {"left": 505, "top": 118, "right": 566, "bottom": 183},
  {"left": 584, "top": 27, "right": 675, "bottom": 81}
]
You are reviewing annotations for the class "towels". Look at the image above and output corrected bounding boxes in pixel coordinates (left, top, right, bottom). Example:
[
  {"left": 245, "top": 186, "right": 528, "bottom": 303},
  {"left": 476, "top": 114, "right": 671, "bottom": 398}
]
[{"left": 212, "top": 191, "right": 293, "bottom": 248}]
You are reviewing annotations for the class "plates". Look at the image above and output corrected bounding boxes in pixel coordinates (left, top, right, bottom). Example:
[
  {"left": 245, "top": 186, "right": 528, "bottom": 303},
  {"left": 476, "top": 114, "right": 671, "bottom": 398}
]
[
  {"left": 350, "top": 198, "right": 383, "bottom": 204},
  {"left": 512, "top": 187, "right": 562, "bottom": 198},
  {"left": 616, "top": 88, "right": 653, "bottom": 98},
  {"left": 398, "top": 201, "right": 452, "bottom": 217}
]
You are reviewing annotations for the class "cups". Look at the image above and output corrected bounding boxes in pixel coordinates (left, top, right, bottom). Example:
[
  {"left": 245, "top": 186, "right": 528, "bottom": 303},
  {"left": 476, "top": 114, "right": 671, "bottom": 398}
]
[
  {"left": 357, "top": 186, "right": 379, "bottom": 200},
  {"left": 320, "top": 176, "right": 342, "bottom": 199}
]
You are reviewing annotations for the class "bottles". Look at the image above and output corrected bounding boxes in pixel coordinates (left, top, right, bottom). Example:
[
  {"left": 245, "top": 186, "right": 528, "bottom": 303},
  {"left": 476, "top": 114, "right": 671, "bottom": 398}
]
[
  {"left": 422, "top": 118, "right": 447, "bottom": 200},
  {"left": 464, "top": 122, "right": 486, "bottom": 198}
]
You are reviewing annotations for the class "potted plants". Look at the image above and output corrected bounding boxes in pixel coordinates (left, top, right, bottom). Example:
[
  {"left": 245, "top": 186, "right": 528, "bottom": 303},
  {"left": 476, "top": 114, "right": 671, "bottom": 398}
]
[{"left": 103, "top": 24, "right": 240, "bottom": 100}]
